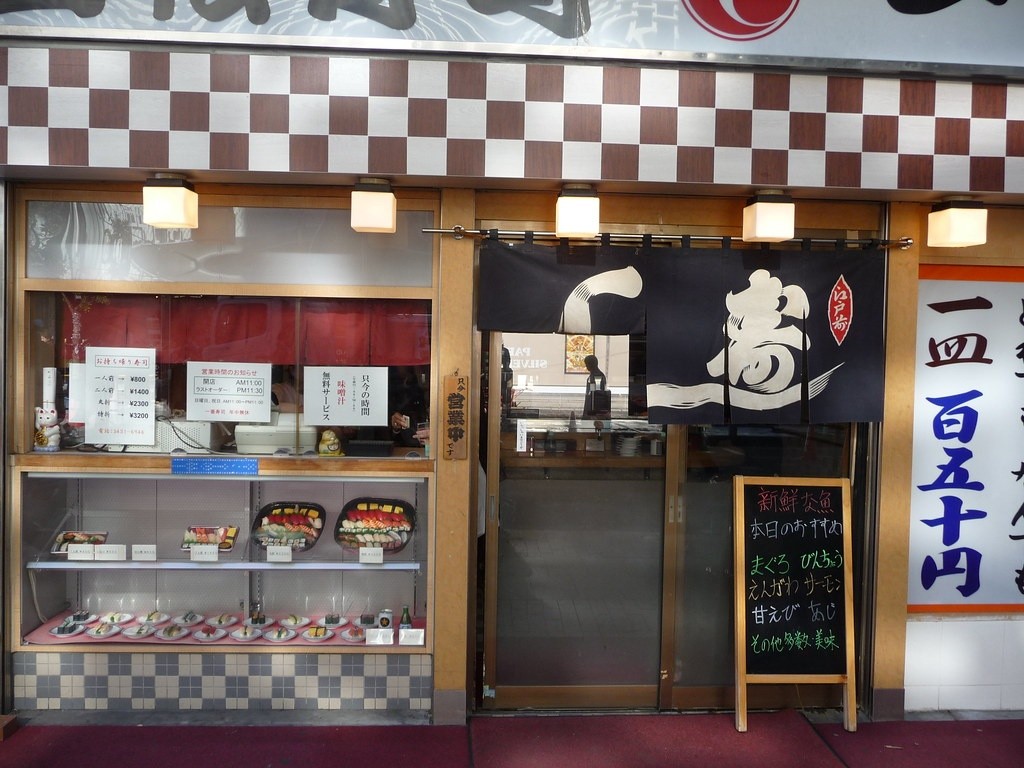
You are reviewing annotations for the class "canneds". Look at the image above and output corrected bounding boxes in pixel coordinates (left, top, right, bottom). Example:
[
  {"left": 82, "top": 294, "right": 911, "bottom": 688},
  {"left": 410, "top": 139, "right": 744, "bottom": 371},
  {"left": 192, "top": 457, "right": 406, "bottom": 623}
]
[{"left": 379, "top": 609, "right": 393, "bottom": 629}]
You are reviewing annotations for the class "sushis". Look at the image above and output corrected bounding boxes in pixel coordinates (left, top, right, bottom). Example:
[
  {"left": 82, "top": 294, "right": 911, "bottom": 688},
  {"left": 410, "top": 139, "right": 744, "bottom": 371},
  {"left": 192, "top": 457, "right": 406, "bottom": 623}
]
[
  {"left": 57, "top": 609, "right": 375, "bottom": 639},
  {"left": 49, "top": 502, "right": 418, "bottom": 553}
]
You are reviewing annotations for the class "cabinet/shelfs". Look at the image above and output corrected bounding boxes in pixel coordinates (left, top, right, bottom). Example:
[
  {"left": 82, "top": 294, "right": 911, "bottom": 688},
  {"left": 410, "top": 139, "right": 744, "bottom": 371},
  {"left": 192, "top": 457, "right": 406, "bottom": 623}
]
[{"left": 9, "top": 446, "right": 436, "bottom": 652}]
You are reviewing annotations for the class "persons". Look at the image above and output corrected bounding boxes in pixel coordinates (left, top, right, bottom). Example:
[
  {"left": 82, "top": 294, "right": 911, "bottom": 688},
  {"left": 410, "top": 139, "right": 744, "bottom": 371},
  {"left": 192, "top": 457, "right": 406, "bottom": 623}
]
[
  {"left": 271, "top": 362, "right": 430, "bottom": 446},
  {"left": 584, "top": 355, "right": 606, "bottom": 395}
]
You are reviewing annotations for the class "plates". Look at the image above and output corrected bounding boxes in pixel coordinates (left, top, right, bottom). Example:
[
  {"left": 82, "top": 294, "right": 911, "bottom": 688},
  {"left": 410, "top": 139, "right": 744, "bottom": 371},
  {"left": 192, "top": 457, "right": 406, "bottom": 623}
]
[
  {"left": 49, "top": 625, "right": 86, "bottom": 638},
  {"left": 230, "top": 628, "right": 262, "bottom": 641},
  {"left": 264, "top": 629, "right": 297, "bottom": 642},
  {"left": 206, "top": 616, "right": 238, "bottom": 628},
  {"left": 121, "top": 626, "right": 155, "bottom": 638},
  {"left": 616, "top": 435, "right": 641, "bottom": 457},
  {"left": 301, "top": 630, "right": 334, "bottom": 642},
  {"left": 251, "top": 502, "right": 326, "bottom": 553},
  {"left": 171, "top": 614, "right": 204, "bottom": 626},
  {"left": 279, "top": 617, "right": 311, "bottom": 629},
  {"left": 317, "top": 618, "right": 349, "bottom": 629},
  {"left": 352, "top": 617, "right": 378, "bottom": 628},
  {"left": 340, "top": 629, "right": 366, "bottom": 642},
  {"left": 137, "top": 613, "right": 170, "bottom": 626},
  {"left": 99, "top": 613, "right": 135, "bottom": 625},
  {"left": 243, "top": 618, "right": 275, "bottom": 629},
  {"left": 84, "top": 626, "right": 121, "bottom": 639},
  {"left": 65, "top": 614, "right": 99, "bottom": 624},
  {"left": 193, "top": 629, "right": 229, "bottom": 642},
  {"left": 334, "top": 497, "right": 416, "bottom": 554},
  {"left": 155, "top": 628, "right": 190, "bottom": 640}
]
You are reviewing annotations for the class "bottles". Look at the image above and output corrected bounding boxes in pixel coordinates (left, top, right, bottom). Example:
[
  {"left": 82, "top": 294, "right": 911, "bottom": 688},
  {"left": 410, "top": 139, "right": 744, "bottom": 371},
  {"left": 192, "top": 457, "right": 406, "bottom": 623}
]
[
  {"left": 398, "top": 606, "right": 412, "bottom": 629},
  {"left": 544, "top": 431, "right": 556, "bottom": 452}
]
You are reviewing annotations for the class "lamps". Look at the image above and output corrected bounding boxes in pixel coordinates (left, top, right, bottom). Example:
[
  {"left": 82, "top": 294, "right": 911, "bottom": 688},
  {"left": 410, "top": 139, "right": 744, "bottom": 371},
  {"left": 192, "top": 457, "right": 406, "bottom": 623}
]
[
  {"left": 742, "top": 191, "right": 796, "bottom": 242},
  {"left": 556, "top": 183, "right": 600, "bottom": 240},
  {"left": 142, "top": 172, "right": 199, "bottom": 230},
  {"left": 350, "top": 178, "right": 396, "bottom": 234},
  {"left": 927, "top": 195, "right": 988, "bottom": 247}
]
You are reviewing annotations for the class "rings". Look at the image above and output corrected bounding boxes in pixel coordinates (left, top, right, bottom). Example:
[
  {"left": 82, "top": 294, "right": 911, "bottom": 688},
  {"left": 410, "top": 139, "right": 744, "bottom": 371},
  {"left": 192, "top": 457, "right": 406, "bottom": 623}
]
[{"left": 395, "top": 423, "right": 396, "bottom": 426}]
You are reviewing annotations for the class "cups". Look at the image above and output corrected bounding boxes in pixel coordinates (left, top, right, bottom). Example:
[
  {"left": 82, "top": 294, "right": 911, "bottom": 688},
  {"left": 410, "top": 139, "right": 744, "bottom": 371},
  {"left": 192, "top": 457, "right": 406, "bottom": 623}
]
[
  {"left": 650, "top": 439, "right": 662, "bottom": 455},
  {"left": 417, "top": 422, "right": 429, "bottom": 445}
]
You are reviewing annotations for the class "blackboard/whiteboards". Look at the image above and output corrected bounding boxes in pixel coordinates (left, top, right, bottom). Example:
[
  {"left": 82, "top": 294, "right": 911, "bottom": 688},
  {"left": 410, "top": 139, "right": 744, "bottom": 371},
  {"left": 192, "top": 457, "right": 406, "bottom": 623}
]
[{"left": 732, "top": 474, "right": 857, "bottom": 683}]
